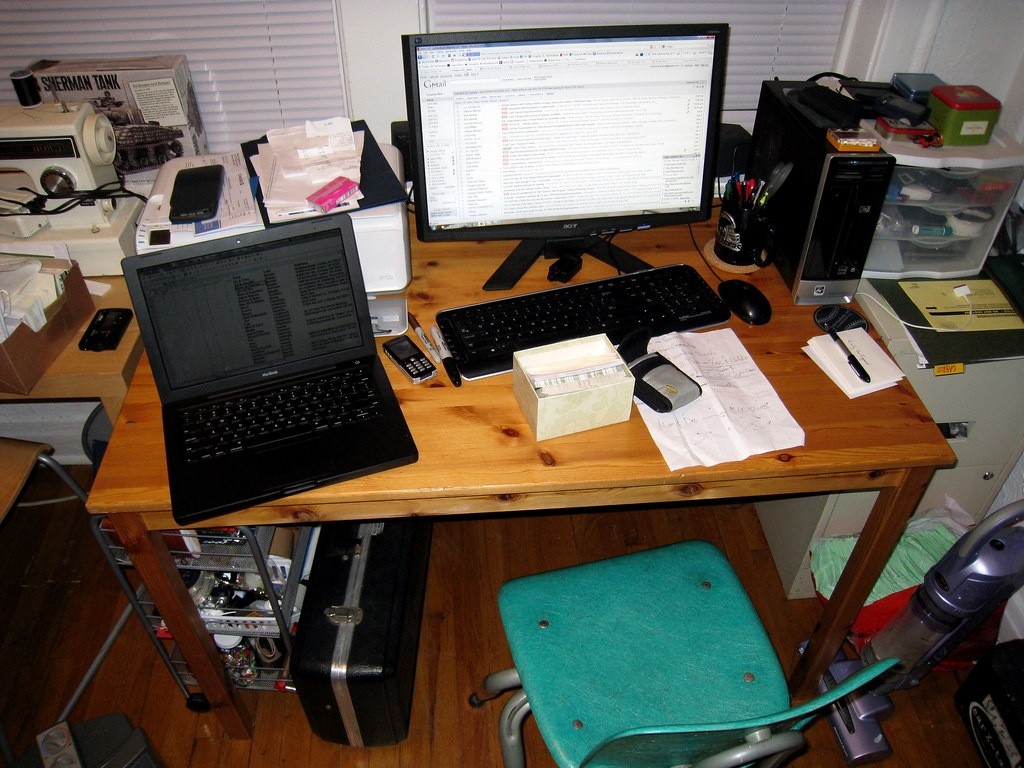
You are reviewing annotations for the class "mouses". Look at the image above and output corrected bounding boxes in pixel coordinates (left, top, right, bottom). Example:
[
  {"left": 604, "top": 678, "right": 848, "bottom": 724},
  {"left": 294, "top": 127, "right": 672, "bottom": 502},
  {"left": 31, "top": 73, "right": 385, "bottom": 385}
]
[{"left": 719, "top": 279, "right": 772, "bottom": 327}]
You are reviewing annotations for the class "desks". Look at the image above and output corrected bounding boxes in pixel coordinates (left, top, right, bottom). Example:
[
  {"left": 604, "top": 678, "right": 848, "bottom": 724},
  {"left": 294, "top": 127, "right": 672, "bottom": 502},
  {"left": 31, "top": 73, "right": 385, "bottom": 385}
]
[{"left": 86, "top": 204, "right": 957, "bottom": 740}]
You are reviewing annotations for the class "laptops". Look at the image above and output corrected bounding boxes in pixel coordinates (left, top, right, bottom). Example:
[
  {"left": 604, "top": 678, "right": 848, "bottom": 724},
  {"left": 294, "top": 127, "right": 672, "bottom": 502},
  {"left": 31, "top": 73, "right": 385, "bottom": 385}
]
[{"left": 120, "top": 213, "right": 419, "bottom": 526}]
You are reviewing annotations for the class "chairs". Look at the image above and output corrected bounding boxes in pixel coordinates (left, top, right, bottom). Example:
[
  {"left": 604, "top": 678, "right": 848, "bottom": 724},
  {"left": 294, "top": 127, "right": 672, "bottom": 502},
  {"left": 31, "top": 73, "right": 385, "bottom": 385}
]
[
  {"left": 0, "top": 435, "right": 209, "bottom": 725},
  {"left": 486, "top": 537, "right": 903, "bottom": 768}
]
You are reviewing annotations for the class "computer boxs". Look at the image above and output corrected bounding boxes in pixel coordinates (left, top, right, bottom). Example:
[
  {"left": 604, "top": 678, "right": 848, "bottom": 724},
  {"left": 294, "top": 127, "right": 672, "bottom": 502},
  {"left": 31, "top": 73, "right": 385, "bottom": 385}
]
[{"left": 752, "top": 78, "right": 896, "bottom": 306}]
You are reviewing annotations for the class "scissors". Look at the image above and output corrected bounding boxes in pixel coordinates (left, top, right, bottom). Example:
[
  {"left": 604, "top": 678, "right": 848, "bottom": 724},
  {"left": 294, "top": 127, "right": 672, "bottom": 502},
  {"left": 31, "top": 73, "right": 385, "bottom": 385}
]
[{"left": 763, "top": 162, "right": 795, "bottom": 199}]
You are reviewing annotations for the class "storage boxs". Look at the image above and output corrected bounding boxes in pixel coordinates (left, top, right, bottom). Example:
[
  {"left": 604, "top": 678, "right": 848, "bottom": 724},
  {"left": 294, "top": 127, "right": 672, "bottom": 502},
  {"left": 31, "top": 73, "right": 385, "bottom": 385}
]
[
  {"left": 7, "top": 712, "right": 161, "bottom": 768},
  {"left": 861, "top": 117, "right": 1024, "bottom": 281},
  {"left": 1, "top": 253, "right": 98, "bottom": 395},
  {"left": 511, "top": 335, "right": 636, "bottom": 444},
  {"left": 133, "top": 144, "right": 411, "bottom": 293},
  {"left": 874, "top": 116, "right": 936, "bottom": 141},
  {"left": 30, "top": 54, "right": 208, "bottom": 182},
  {"left": 926, "top": 85, "right": 1001, "bottom": 147},
  {"left": 890, "top": 74, "right": 945, "bottom": 102}
]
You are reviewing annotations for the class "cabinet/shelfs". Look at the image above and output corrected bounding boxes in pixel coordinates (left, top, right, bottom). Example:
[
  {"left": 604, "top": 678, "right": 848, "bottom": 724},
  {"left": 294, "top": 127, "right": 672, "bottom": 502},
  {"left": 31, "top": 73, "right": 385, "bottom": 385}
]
[
  {"left": 83, "top": 508, "right": 302, "bottom": 714},
  {"left": 755, "top": 282, "right": 1024, "bottom": 600}
]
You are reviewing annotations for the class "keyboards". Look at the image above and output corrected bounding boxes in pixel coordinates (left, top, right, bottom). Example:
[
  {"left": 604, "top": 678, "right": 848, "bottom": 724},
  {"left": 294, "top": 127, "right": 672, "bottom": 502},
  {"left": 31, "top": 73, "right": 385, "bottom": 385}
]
[{"left": 434, "top": 264, "right": 734, "bottom": 381}]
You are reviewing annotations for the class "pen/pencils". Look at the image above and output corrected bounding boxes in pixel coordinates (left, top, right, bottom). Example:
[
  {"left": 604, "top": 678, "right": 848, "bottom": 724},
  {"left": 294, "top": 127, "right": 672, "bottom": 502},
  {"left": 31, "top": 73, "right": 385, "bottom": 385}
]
[
  {"left": 408, "top": 311, "right": 440, "bottom": 363},
  {"left": 724, "top": 172, "right": 769, "bottom": 213},
  {"left": 824, "top": 321, "right": 871, "bottom": 383}
]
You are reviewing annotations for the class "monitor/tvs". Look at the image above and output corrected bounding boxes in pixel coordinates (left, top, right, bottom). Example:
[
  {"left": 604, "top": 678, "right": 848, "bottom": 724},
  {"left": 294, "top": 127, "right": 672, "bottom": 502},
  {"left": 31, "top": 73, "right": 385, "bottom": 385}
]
[{"left": 400, "top": 22, "right": 732, "bottom": 291}]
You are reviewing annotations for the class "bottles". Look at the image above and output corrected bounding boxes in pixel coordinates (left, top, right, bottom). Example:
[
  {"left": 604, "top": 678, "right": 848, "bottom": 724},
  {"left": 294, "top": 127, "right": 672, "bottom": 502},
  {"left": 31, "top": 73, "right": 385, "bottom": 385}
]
[{"left": 214, "top": 633, "right": 260, "bottom": 687}]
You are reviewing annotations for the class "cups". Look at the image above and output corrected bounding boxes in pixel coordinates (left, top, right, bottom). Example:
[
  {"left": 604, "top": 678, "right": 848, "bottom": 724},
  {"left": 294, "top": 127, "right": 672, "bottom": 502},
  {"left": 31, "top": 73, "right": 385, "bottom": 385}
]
[{"left": 713, "top": 195, "right": 775, "bottom": 268}]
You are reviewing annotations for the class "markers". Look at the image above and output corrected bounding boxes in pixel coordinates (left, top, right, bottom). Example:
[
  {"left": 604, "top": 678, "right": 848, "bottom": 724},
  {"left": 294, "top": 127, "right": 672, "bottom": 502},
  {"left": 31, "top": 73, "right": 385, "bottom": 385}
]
[{"left": 430, "top": 326, "right": 462, "bottom": 387}]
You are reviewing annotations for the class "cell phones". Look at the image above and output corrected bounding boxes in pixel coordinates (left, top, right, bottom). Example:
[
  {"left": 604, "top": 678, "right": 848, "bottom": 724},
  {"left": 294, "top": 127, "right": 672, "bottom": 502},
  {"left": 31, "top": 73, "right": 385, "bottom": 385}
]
[
  {"left": 381, "top": 335, "right": 439, "bottom": 385},
  {"left": 77, "top": 307, "right": 132, "bottom": 352},
  {"left": 168, "top": 164, "right": 226, "bottom": 222}
]
